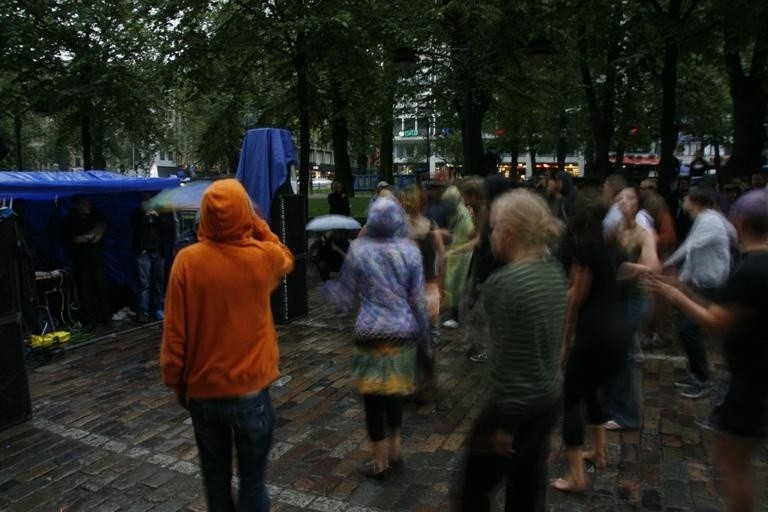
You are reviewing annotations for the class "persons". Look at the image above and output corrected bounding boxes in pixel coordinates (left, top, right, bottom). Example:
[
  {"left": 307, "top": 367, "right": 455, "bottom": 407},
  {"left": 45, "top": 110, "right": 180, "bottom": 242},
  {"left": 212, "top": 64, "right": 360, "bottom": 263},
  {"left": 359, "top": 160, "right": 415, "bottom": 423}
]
[
  {"left": 65, "top": 196, "right": 107, "bottom": 311},
  {"left": 129, "top": 192, "right": 171, "bottom": 324},
  {"left": 159, "top": 178, "right": 295, "bottom": 511}
]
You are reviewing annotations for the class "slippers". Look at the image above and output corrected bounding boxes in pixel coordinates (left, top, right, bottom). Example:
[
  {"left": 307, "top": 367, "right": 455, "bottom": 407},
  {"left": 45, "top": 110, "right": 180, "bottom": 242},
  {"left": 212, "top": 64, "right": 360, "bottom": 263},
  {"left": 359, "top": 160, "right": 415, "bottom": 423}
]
[{"left": 606, "top": 419, "right": 643, "bottom": 433}]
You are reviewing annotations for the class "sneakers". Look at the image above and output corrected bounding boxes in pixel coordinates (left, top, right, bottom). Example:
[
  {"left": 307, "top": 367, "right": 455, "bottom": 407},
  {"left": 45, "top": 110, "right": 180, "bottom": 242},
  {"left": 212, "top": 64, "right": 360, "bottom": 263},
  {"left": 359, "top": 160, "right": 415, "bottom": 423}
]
[
  {"left": 674, "top": 373, "right": 701, "bottom": 387},
  {"left": 681, "top": 382, "right": 716, "bottom": 398},
  {"left": 470, "top": 352, "right": 491, "bottom": 362},
  {"left": 442, "top": 319, "right": 460, "bottom": 329}
]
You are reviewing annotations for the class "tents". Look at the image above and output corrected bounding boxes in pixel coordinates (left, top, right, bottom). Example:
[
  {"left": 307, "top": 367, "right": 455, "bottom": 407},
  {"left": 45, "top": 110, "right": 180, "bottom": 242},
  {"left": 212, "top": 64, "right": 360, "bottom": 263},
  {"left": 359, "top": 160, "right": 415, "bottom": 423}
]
[{"left": 0, "top": 164, "right": 181, "bottom": 308}]
